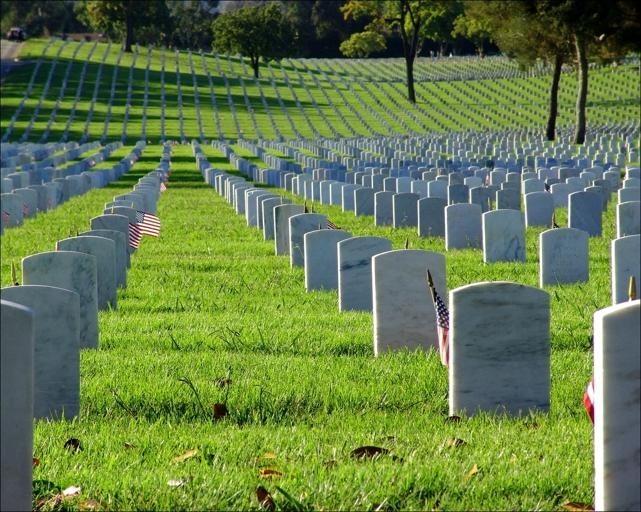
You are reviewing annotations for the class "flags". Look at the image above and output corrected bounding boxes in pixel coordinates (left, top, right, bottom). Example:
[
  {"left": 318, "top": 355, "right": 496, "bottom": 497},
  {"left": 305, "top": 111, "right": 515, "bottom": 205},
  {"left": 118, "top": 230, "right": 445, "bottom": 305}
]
[
  {"left": 435, "top": 293, "right": 451, "bottom": 364},
  {"left": 129, "top": 222, "right": 141, "bottom": 249},
  {"left": 137, "top": 212, "right": 160, "bottom": 237}
]
